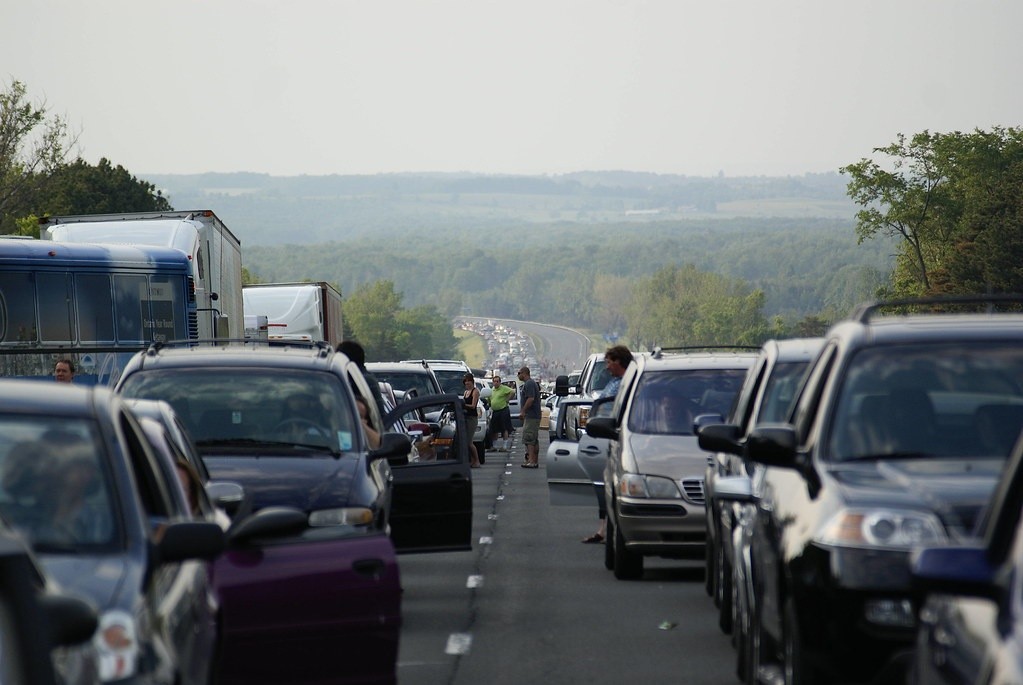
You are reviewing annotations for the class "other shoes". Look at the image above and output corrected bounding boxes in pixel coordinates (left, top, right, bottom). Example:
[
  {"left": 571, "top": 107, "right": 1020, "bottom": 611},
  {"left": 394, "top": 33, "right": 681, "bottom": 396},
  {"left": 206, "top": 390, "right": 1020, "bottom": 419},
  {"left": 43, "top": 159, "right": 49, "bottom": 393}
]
[
  {"left": 521, "top": 463, "right": 538, "bottom": 469},
  {"left": 471, "top": 463, "right": 481, "bottom": 468},
  {"left": 580, "top": 533, "right": 603, "bottom": 543},
  {"left": 498, "top": 447, "right": 508, "bottom": 452},
  {"left": 487, "top": 448, "right": 497, "bottom": 452}
]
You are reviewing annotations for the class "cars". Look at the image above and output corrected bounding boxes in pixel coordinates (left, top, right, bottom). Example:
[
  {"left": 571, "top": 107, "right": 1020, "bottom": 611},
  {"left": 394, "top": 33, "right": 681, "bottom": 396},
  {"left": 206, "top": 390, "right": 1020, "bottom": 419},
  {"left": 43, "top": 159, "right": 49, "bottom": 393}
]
[
  {"left": 694, "top": 339, "right": 834, "bottom": 631},
  {"left": 0, "top": 322, "right": 558, "bottom": 685},
  {"left": 541, "top": 347, "right": 681, "bottom": 505},
  {"left": 907, "top": 432, "right": 1023, "bottom": 685}
]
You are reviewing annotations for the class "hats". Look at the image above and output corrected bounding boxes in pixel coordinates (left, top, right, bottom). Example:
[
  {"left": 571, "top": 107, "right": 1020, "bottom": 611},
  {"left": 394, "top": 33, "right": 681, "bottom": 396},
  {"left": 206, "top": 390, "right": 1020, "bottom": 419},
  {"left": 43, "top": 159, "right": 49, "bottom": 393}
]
[{"left": 463, "top": 373, "right": 474, "bottom": 385}]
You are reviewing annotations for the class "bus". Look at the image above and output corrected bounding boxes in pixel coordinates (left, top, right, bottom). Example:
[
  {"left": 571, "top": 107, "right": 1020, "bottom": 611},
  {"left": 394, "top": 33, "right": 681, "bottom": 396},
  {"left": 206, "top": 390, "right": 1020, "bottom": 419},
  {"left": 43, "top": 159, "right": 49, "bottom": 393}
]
[{"left": 0, "top": 235, "right": 200, "bottom": 387}]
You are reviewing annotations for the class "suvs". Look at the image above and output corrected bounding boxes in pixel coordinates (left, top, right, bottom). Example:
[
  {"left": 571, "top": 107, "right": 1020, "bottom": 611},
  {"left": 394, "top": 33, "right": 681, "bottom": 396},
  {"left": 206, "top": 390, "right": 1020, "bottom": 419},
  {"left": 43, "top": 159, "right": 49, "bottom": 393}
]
[
  {"left": 584, "top": 347, "right": 768, "bottom": 585},
  {"left": 109, "top": 339, "right": 411, "bottom": 534},
  {"left": 744, "top": 293, "right": 1022, "bottom": 685}
]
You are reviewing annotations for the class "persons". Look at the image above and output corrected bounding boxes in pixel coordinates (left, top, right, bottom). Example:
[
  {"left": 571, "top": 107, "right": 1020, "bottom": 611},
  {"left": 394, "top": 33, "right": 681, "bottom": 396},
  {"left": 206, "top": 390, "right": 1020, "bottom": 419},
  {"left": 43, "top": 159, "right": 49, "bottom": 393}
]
[
  {"left": 484, "top": 375, "right": 516, "bottom": 451},
  {"left": 335, "top": 398, "right": 379, "bottom": 450},
  {"left": 336, "top": 341, "right": 385, "bottom": 423},
  {"left": 580, "top": 346, "right": 636, "bottom": 543},
  {"left": 516, "top": 367, "right": 542, "bottom": 469},
  {"left": 450, "top": 373, "right": 479, "bottom": 469},
  {"left": 54, "top": 358, "right": 77, "bottom": 385},
  {"left": 31, "top": 430, "right": 166, "bottom": 552}
]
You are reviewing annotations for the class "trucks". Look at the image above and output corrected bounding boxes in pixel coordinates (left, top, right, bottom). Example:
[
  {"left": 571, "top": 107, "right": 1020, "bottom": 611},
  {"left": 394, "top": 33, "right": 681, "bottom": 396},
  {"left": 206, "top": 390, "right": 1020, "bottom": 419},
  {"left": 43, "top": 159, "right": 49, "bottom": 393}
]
[
  {"left": 242, "top": 281, "right": 344, "bottom": 353},
  {"left": 39, "top": 209, "right": 246, "bottom": 350}
]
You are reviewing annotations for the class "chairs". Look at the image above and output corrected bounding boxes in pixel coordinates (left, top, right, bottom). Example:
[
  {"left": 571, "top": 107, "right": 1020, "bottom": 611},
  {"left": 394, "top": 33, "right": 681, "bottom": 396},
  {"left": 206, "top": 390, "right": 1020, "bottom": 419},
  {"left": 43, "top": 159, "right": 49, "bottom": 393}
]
[
  {"left": 276, "top": 392, "right": 334, "bottom": 432},
  {"left": 970, "top": 403, "right": 1022, "bottom": 455},
  {"left": 200, "top": 411, "right": 259, "bottom": 439},
  {"left": 862, "top": 383, "right": 948, "bottom": 454}
]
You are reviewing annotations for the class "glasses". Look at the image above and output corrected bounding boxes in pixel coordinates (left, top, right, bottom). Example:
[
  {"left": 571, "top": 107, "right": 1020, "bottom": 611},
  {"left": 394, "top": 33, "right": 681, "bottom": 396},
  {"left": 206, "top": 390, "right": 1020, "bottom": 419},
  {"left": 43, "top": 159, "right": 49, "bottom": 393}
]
[{"left": 518, "top": 372, "right": 521, "bottom": 375}]
